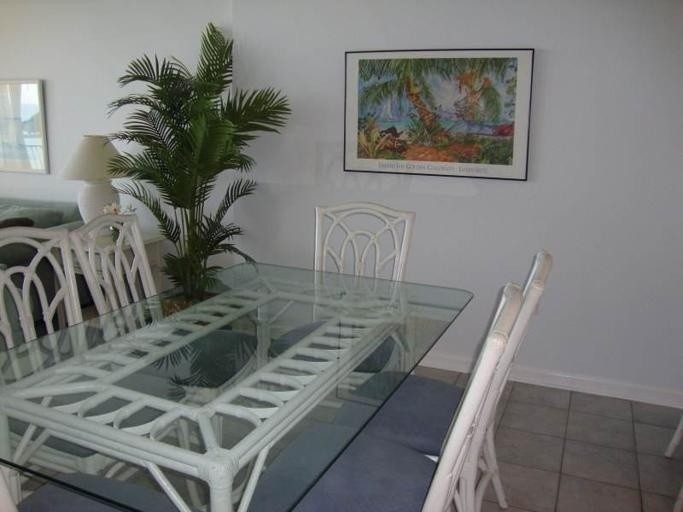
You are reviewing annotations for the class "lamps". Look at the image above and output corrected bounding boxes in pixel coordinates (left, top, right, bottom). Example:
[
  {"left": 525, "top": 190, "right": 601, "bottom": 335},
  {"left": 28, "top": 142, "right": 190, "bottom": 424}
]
[{"left": 57, "top": 135, "right": 135, "bottom": 239}]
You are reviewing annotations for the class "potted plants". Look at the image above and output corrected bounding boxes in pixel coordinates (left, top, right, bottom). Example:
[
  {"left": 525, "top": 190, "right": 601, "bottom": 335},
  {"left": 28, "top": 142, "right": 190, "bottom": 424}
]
[{"left": 102, "top": 21, "right": 291, "bottom": 382}]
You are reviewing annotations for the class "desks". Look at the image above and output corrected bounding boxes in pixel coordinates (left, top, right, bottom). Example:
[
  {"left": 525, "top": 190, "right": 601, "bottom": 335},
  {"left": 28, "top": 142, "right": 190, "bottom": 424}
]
[{"left": 0, "top": 263, "right": 475, "bottom": 511}]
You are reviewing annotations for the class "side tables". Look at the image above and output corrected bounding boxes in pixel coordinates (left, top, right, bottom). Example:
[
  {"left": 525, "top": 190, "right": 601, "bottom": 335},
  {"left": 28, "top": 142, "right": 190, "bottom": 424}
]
[{"left": 47, "top": 230, "right": 168, "bottom": 341}]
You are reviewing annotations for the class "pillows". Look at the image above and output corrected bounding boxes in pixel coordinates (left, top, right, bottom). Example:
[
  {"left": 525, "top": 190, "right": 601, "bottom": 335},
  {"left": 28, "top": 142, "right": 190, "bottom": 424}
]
[{"left": 0, "top": 203, "right": 62, "bottom": 229}]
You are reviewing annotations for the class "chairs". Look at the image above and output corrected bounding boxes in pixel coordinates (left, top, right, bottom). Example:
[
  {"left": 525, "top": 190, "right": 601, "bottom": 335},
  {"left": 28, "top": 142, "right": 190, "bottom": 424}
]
[
  {"left": 315, "top": 203, "right": 416, "bottom": 282},
  {"left": 235, "top": 250, "right": 555, "bottom": 510},
  {"left": 0, "top": 227, "right": 185, "bottom": 511}
]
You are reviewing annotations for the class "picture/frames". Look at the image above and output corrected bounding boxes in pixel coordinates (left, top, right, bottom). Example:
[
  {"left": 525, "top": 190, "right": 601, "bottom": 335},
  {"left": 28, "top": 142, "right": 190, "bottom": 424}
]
[
  {"left": 0, "top": 77, "right": 52, "bottom": 176},
  {"left": 340, "top": 47, "right": 535, "bottom": 181}
]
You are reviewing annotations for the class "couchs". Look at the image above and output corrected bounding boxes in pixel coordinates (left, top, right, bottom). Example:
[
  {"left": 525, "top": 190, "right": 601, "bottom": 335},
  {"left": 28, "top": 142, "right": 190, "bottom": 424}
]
[{"left": 0, "top": 198, "right": 105, "bottom": 337}]
